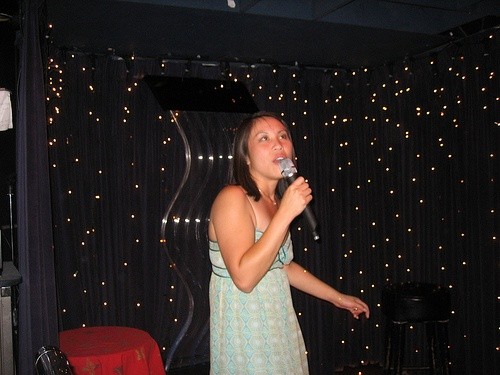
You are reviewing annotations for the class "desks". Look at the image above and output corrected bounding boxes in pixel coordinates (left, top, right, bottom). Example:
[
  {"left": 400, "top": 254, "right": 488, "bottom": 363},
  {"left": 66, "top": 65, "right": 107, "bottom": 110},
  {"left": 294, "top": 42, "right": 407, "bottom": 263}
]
[{"left": 57, "top": 326, "right": 166, "bottom": 375}]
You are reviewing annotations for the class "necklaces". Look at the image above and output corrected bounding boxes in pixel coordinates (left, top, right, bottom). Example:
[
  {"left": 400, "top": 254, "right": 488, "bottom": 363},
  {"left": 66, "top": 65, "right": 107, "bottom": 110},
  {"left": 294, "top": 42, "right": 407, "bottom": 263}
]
[{"left": 258, "top": 186, "right": 277, "bottom": 205}]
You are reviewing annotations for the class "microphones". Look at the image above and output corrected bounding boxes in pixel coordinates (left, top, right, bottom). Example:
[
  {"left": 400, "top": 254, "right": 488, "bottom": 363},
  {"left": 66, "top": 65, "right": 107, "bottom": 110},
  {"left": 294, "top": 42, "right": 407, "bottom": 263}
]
[{"left": 279, "top": 157, "right": 320, "bottom": 241}]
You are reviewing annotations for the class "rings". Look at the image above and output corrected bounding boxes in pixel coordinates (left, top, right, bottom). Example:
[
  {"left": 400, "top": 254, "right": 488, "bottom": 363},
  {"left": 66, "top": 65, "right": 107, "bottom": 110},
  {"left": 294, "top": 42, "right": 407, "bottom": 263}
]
[{"left": 354, "top": 308, "right": 359, "bottom": 311}]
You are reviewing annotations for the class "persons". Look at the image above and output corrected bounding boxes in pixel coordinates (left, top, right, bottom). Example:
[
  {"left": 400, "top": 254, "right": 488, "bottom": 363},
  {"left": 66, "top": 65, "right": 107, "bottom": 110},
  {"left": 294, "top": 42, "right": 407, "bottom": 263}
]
[{"left": 208, "top": 111, "right": 370, "bottom": 375}]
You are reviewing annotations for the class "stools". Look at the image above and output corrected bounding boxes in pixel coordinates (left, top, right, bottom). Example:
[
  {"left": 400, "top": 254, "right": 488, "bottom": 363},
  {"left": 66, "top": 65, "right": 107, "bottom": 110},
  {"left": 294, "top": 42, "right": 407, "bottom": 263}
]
[{"left": 383, "top": 281, "right": 452, "bottom": 375}]
